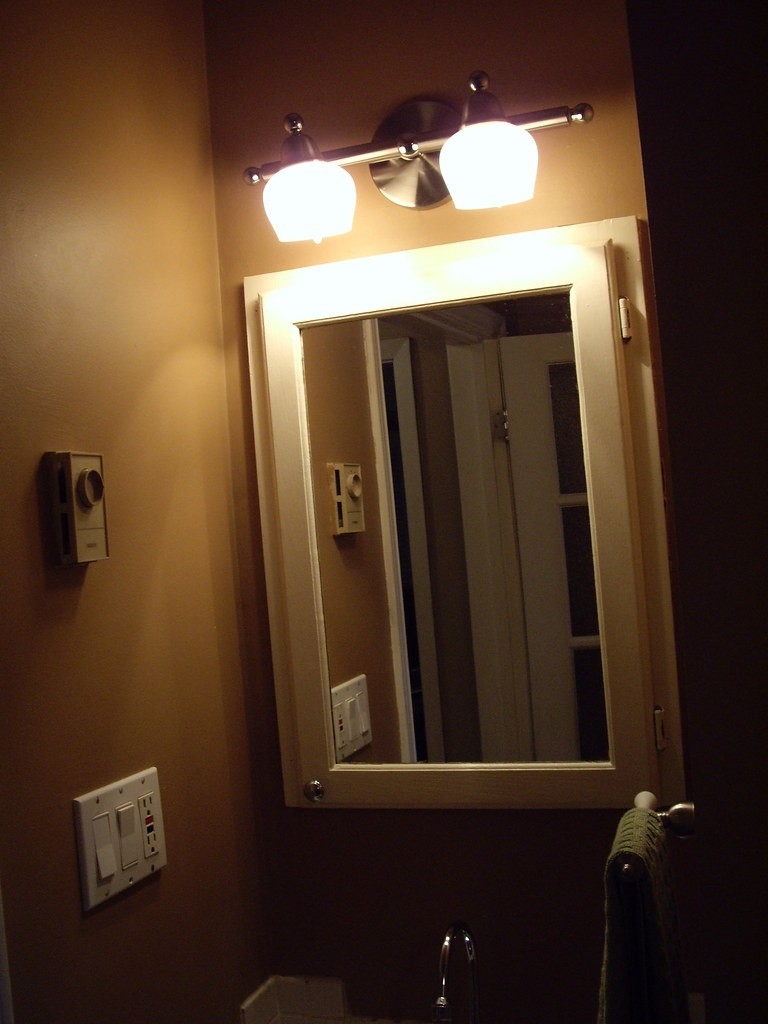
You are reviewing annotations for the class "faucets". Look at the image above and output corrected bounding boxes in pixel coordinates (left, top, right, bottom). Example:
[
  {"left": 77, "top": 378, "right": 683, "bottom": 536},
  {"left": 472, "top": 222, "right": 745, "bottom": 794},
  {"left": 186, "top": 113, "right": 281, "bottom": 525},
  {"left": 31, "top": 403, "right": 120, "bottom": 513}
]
[{"left": 428, "top": 924, "right": 487, "bottom": 1022}]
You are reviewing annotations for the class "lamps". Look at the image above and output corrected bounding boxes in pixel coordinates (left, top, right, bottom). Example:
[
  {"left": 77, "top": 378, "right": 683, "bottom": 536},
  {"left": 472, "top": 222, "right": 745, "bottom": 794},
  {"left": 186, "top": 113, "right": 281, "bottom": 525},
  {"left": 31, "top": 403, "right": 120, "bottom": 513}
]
[
  {"left": 440, "top": 69, "right": 541, "bottom": 211},
  {"left": 264, "top": 113, "right": 354, "bottom": 243}
]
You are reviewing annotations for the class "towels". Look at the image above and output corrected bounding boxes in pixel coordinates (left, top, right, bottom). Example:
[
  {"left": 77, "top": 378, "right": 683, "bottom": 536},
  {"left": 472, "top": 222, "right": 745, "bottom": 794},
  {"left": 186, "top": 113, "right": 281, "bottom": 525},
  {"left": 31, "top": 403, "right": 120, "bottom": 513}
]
[{"left": 595, "top": 807, "right": 691, "bottom": 1024}]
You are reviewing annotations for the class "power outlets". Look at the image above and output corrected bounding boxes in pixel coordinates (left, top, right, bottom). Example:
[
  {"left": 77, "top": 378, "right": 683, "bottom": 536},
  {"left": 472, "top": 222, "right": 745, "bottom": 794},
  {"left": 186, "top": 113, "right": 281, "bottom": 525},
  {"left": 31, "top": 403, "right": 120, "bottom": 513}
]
[{"left": 332, "top": 673, "right": 374, "bottom": 764}]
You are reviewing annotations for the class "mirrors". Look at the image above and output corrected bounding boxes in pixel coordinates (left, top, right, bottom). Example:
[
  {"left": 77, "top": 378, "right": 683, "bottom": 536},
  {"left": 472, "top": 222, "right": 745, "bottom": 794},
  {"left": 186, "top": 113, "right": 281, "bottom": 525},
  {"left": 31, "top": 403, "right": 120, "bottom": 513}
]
[{"left": 257, "top": 240, "right": 660, "bottom": 808}]
